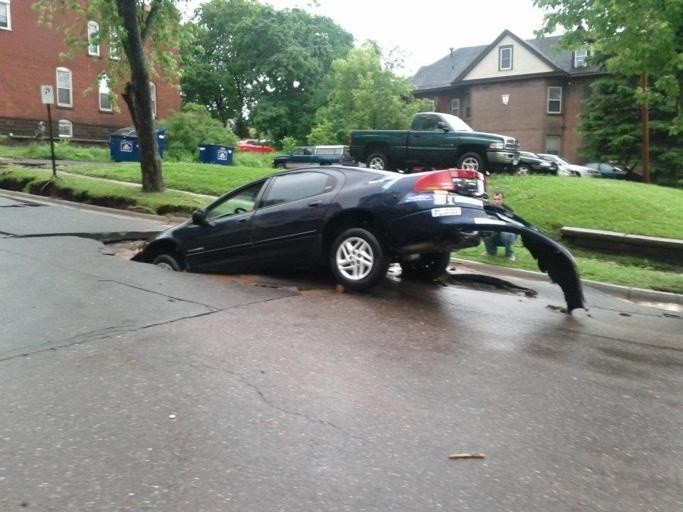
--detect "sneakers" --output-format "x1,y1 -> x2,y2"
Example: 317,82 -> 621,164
504,256 -> 516,261
481,251 -> 497,257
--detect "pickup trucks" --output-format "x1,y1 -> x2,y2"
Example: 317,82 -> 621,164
349,113 -> 519,172
274,146 -> 356,168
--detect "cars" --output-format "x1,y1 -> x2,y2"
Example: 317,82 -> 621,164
238,139 -> 276,152
519,151 -> 638,181
130,167 -> 585,313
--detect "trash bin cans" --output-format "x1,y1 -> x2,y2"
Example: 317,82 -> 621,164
197,143 -> 236,166
110,127 -> 167,163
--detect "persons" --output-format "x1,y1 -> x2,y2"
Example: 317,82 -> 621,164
481,191 -> 517,260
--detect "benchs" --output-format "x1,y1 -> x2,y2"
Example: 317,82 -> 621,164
560,226 -> 683,268
